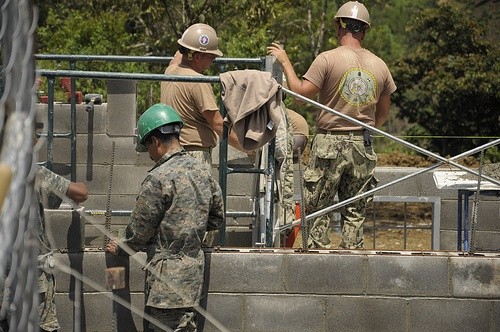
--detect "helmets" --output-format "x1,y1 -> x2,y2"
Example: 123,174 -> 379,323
333,0 -> 369,28
135,102 -> 184,152
176,22 -> 224,58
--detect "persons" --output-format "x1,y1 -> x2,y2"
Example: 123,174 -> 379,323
267,2 -> 399,248
0,119 -> 90,332
279,90 -> 310,249
103,104 -> 225,332
160,22 -> 256,249
